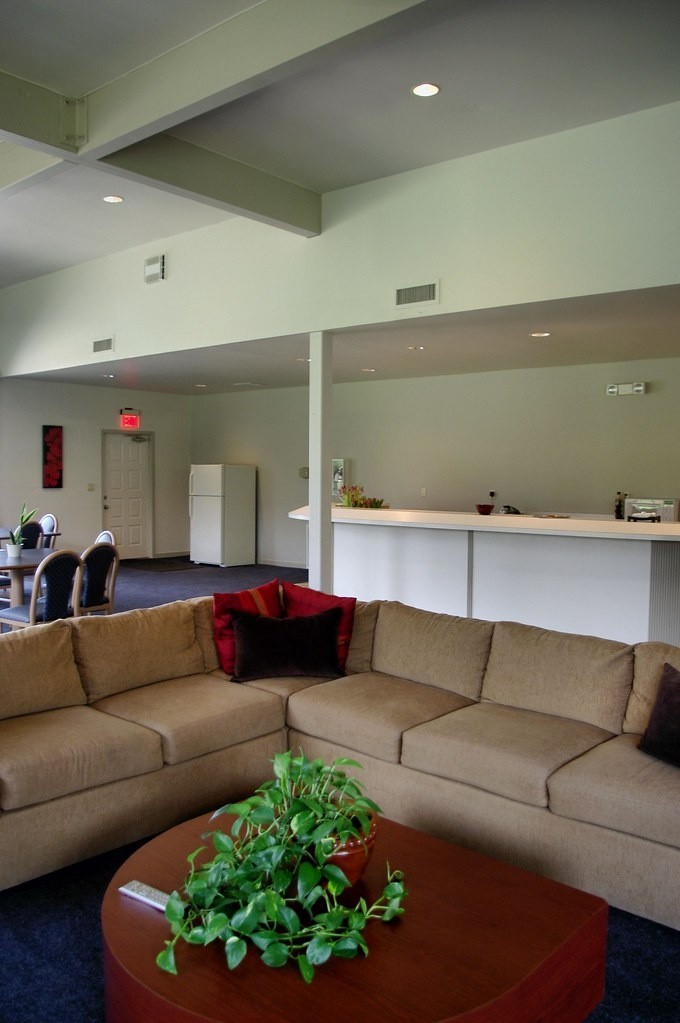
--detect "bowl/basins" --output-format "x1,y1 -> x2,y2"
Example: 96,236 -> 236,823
475,504 -> 494,515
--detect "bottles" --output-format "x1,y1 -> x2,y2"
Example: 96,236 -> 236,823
614,491 -> 624,519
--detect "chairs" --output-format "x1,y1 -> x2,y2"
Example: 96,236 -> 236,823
0,513 -> 120,633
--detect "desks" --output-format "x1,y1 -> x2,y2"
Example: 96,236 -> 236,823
101,775 -> 608,1023
0,549 -> 61,632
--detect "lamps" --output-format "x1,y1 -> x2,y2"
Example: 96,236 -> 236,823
605,381 -> 645,396
410,81 -> 441,97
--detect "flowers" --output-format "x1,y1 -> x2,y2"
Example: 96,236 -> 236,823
335,482 -> 384,509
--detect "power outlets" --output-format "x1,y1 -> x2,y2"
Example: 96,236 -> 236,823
489,490 -> 495,500
420,488 -> 426,497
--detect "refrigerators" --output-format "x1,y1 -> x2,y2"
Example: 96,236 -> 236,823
188,464 -> 256,568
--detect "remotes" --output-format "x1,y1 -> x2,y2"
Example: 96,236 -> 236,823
117,879 -> 170,912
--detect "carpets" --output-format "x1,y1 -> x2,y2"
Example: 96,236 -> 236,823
120,557 -> 202,573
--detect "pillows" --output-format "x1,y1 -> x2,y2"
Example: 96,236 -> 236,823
636,662 -> 680,770
213,577 -> 358,683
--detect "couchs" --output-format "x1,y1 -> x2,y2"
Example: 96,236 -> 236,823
0,584 -> 680,931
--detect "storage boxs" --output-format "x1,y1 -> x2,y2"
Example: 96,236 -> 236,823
625,498 -> 680,521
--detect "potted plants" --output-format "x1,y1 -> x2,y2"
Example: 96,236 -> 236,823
5,503 -> 37,558
154,743 -> 409,978
